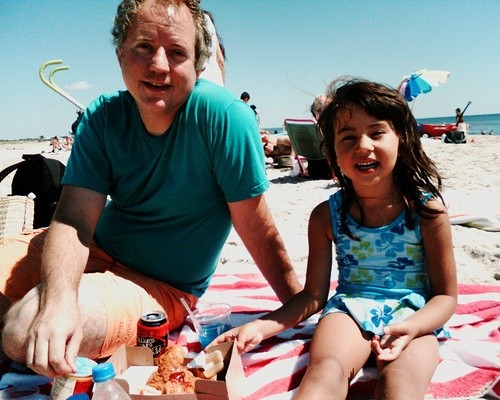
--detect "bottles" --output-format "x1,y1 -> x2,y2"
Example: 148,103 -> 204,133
91,362 -> 133,400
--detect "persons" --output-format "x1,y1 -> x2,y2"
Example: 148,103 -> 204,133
0,0 -> 303,379
442,107 -> 467,144
481,129 -> 494,135
47,135 -> 74,153
239,91 -> 297,172
204,76 -> 459,400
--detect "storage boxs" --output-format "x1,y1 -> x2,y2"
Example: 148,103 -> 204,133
106,338 -> 246,400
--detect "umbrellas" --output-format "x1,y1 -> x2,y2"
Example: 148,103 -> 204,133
397,70 -> 453,102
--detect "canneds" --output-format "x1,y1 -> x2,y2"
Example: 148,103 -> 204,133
50,356 -> 99,400
136,311 -> 168,359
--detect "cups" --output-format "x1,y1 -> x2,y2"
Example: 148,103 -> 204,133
186,303 -> 231,350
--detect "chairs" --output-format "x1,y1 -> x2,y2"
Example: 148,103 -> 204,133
284,119 -> 338,182
